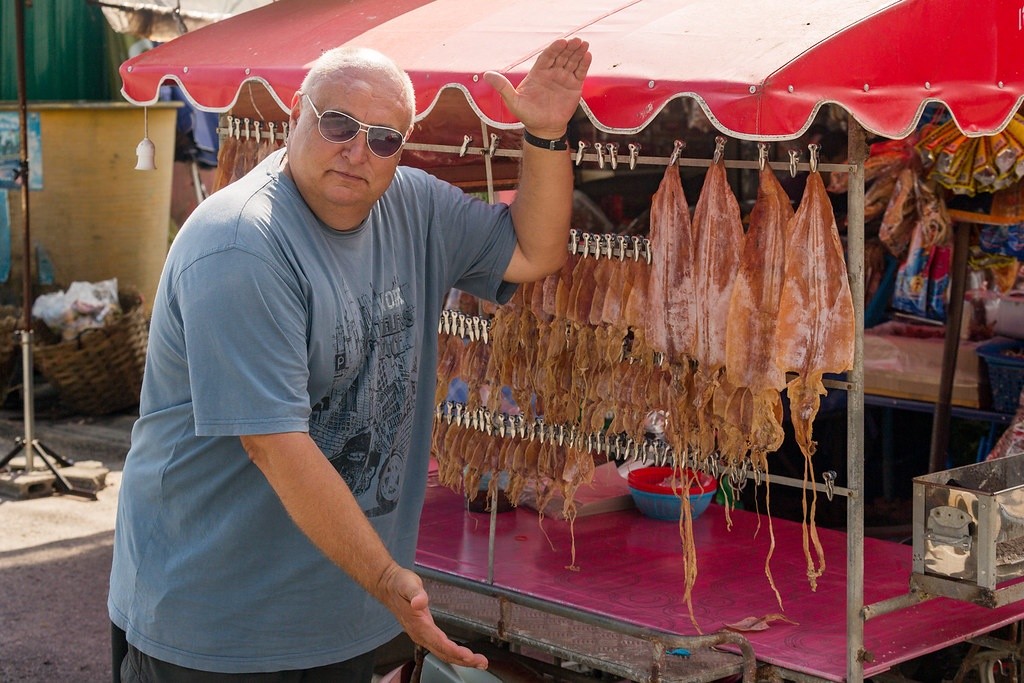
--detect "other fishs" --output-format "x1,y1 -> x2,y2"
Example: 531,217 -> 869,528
429,253 -> 786,573
210,135 -> 288,195
721,612 -> 800,632
689,157 -> 746,464
641,160 -> 705,636
723,161 -> 796,611
768,169 -> 856,593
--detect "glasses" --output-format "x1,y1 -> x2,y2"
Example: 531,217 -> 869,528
300,92 -> 408,158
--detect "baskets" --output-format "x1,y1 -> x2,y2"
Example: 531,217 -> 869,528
32,285 -> 149,411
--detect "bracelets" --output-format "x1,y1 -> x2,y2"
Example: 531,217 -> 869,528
523,125 -> 568,151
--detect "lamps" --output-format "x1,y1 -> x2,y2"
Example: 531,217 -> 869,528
132,105 -> 158,173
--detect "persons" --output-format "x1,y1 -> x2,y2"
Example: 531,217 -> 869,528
106,36 -> 593,682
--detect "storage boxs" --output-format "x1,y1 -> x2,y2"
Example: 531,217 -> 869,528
27,284 -> 148,415
846,318 -> 1000,410
973,340 -> 1024,415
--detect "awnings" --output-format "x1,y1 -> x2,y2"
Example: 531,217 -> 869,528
116,1 -> 1024,141
